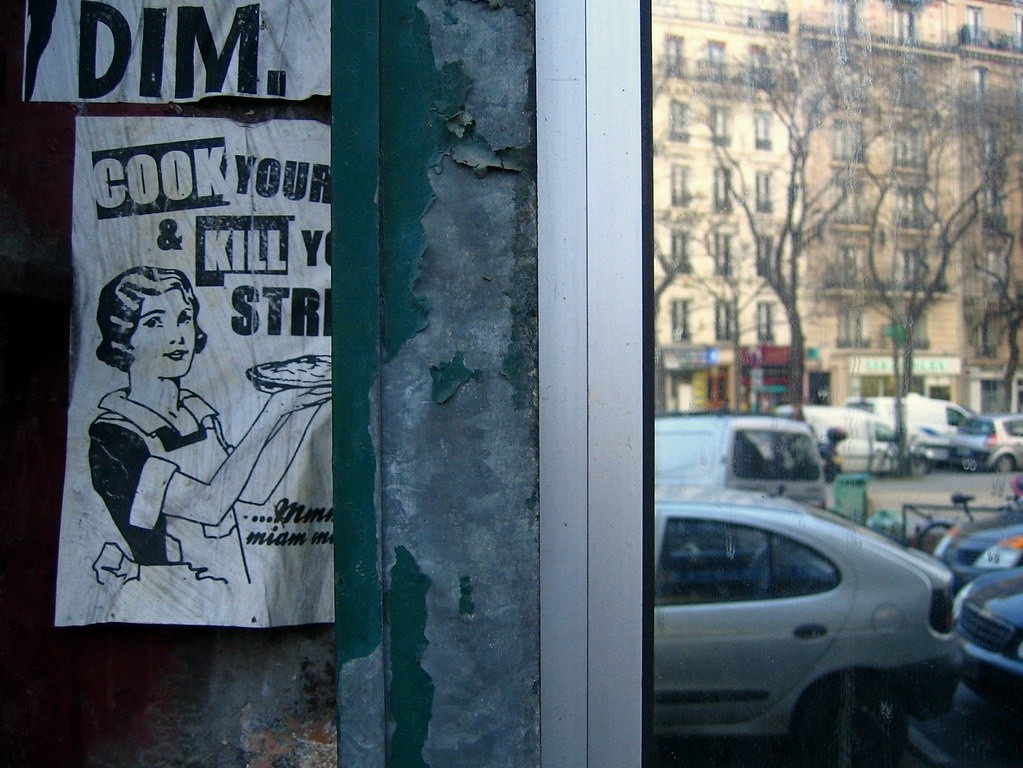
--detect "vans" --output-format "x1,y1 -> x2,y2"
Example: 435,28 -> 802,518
655,413 -> 827,510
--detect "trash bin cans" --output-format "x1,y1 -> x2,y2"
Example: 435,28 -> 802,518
865,508 -> 903,542
831,473 -> 870,525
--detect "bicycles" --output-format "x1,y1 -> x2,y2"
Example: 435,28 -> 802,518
917,489 -> 1023,553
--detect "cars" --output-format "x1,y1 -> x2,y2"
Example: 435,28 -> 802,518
932,506 -> 1023,716
655,481 -> 964,768
773,391 -> 1023,476
